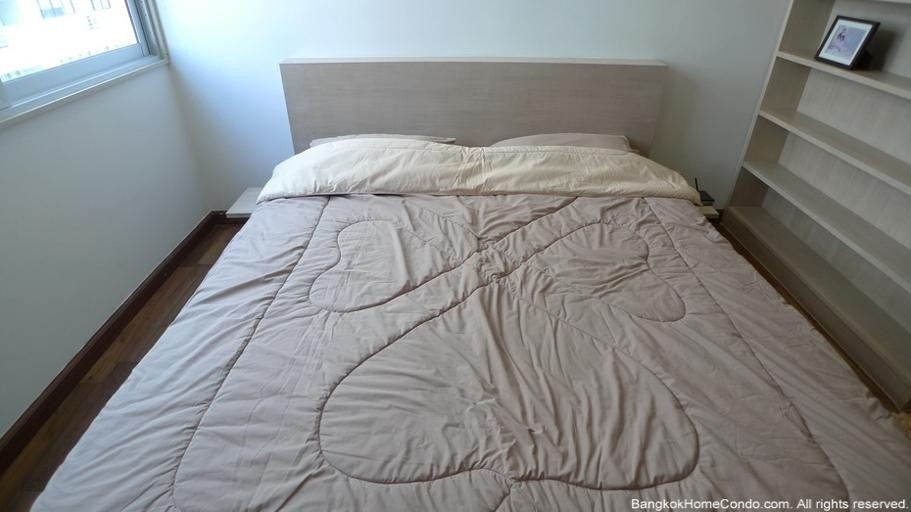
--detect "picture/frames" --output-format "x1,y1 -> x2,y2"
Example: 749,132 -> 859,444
815,13 -> 882,70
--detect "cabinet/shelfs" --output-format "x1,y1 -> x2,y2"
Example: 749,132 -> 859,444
720,0 -> 908,415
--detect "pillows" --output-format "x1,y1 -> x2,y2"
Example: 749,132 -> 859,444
308,132 -> 456,145
486,133 -> 643,155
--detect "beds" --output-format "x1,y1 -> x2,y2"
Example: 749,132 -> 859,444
22,58 -> 909,512
